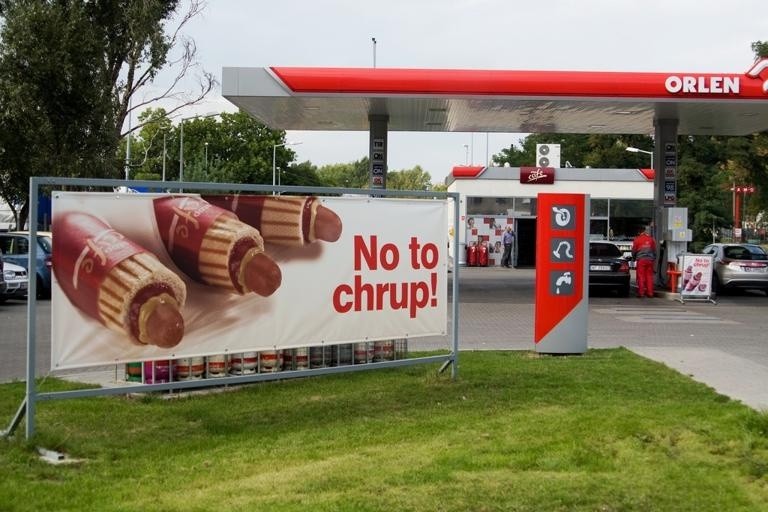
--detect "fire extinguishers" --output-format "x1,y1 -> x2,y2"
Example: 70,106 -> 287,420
479,242 -> 488,266
468,241 -> 477,267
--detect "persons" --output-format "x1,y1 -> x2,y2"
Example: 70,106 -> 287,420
494,241 -> 501,253
480,240 -> 488,247
467,218 -> 476,229
489,217 -> 501,230
500,224 -> 516,269
478,235 -> 482,244
632,225 -> 657,299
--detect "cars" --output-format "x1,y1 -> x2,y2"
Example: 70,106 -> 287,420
0,230 -> 52,300
588,243 -> 632,298
696,243 -> 767,296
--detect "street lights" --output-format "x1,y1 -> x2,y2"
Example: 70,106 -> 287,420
272,141 -> 303,195
625,147 -> 653,169
179,111 -> 220,194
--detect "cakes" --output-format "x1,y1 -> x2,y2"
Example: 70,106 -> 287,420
97,197 -> 343,349
